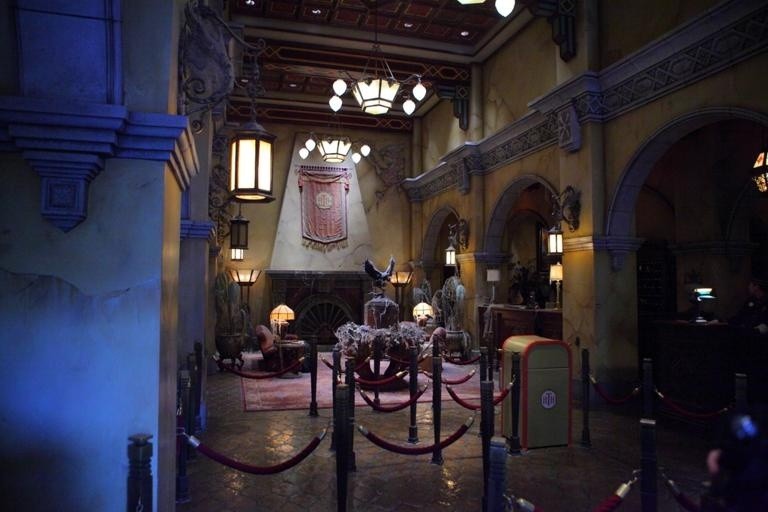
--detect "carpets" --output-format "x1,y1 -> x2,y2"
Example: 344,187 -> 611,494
238,350 -> 506,413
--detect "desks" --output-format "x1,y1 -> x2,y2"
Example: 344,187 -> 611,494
476,303 -> 564,374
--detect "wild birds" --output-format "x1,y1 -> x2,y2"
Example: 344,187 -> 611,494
364,255 -> 396,294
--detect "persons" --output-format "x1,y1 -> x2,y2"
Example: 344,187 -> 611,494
724,274 -> 767,331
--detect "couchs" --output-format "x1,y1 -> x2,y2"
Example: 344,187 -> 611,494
254,324 -> 314,374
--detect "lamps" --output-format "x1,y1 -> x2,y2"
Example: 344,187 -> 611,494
328,1 -> 427,116
389,270 -> 415,322
549,263 -> 563,310
298,68 -> 372,164
750,145 -> 768,197
444,218 -> 468,267
228,268 -> 262,310
546,185 -> 582,256
486,268 -> 502,304
177,0 -> 278,262
270,302 -> 295,338
412,299 -> 437,325
